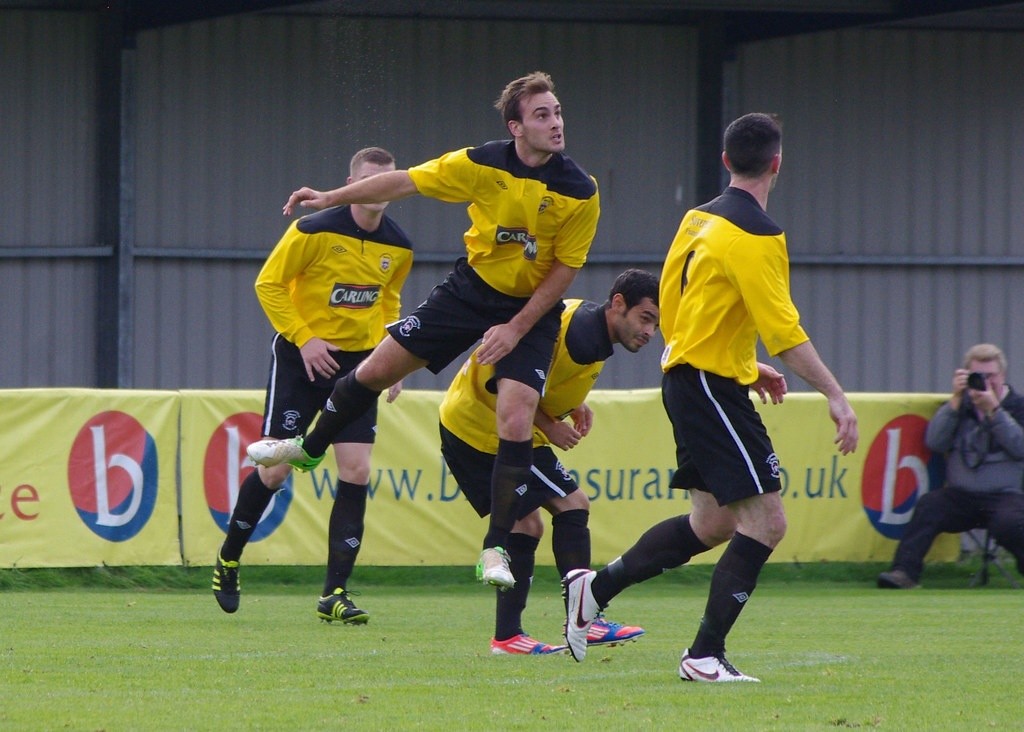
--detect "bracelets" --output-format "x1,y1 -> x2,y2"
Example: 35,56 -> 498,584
987,405 -> 1003,417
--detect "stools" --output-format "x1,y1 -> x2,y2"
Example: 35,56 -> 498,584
965,526 -> 1019,589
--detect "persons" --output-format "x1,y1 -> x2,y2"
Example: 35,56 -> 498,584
246,72 -> 600,589
212,147 -> 413,626
878,343 -> 1024,589
559,112 -> 859,683
439,268 -> 660,655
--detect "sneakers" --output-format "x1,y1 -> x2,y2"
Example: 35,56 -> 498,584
490,632 -> 569,656
678,647 -> 760,683
564,615 -> 646,647
212,546 -> 241,614
246,435 -> 327,473
315,586 -> 370,627
561,568 -> 609,662
475,546 -> 517,592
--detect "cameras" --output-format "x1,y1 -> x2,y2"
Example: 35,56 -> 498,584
967,370 -> 988,392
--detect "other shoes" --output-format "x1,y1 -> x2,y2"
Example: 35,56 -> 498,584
878,568 -> 916,589
